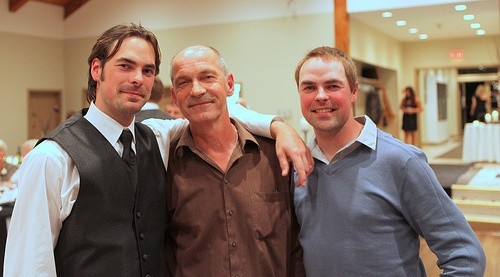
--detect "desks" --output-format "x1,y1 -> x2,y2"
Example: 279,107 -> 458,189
461,122 -> 500,166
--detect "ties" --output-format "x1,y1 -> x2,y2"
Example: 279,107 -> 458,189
119,129 -> 138,197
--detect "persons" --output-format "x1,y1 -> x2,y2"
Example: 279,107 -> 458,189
290,46 -> 486,277
401,87 -> 421,146
469,81 -> 500,123
0,21 -> 314,277
